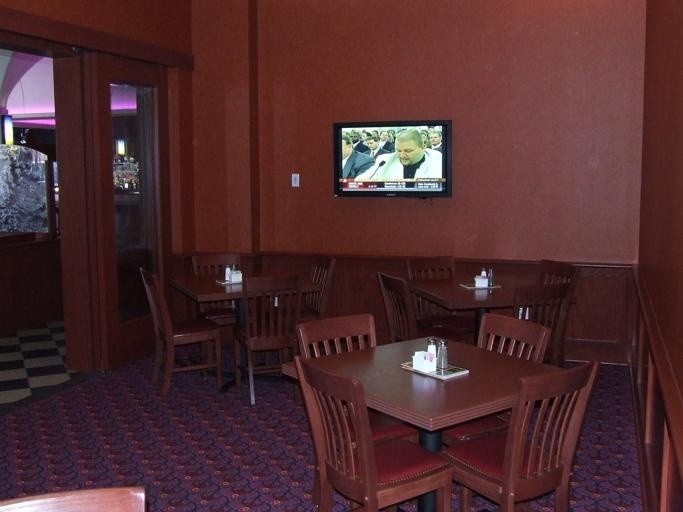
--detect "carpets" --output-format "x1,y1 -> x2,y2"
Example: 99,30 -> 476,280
0,342 -> 644,512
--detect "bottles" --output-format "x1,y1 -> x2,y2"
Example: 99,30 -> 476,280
488,267 -> 494,287
232,264 -> 237,275
437,340 -> 449,370
426,338 -> 437,359
224,264 -> 230,282
481,269 -> 486,276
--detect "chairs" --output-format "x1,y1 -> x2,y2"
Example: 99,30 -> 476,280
293,255 -> 334,361
139,266 -> 222,396
438,312 -> 550,446
377,273 -> 465,363
476,279 -> 576,357
232,271 -> 303,405
191,254 -> 243,325
438,357 -> 601,512
537,258 -> 582,336
407,257 -> 472,334
292,356 -> 453,512
293,313 -> 420,448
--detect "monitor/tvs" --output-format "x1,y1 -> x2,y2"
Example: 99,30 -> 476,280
332,120 -> 453,198
